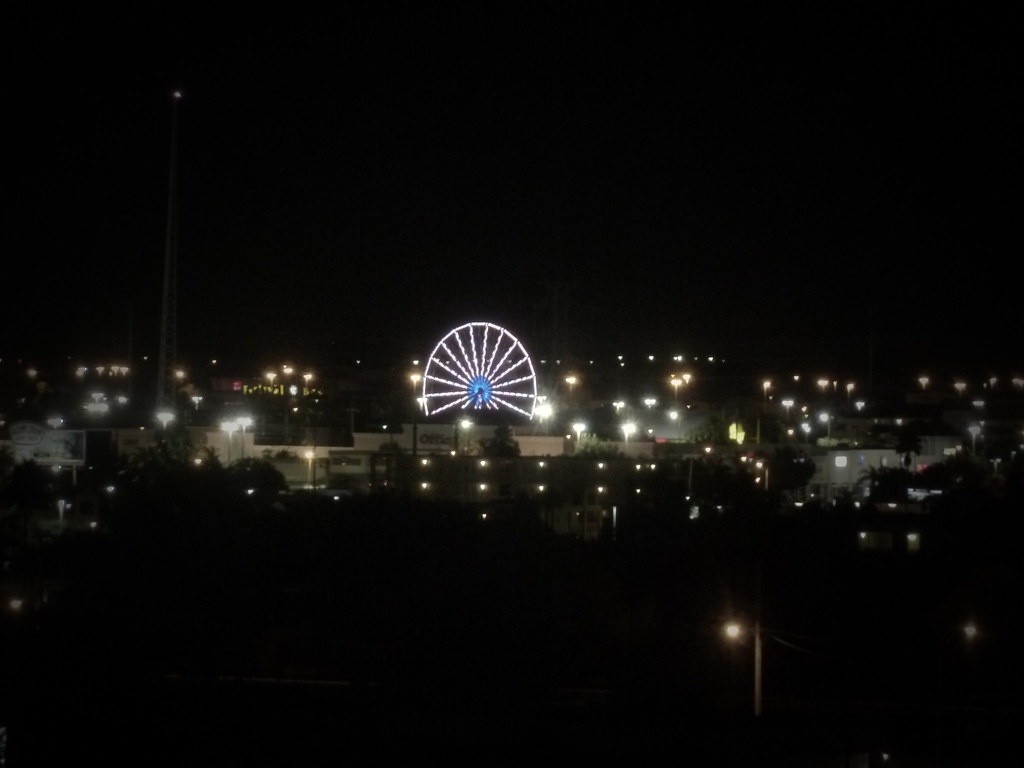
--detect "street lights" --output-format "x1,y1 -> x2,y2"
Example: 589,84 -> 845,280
623,422 -> 638,449
955,381 -> 966,416
222,421 -> 234,466
967,424 -> 979,454
818,412 -> 832,442
723,621 -> 762,720
238,418 -> 251,461
991,457 -> 1002,477
671,376 -> 683,411
568,374 -> 578,397
761,380 -> 773,412
408,372 -> 420,454
782,399 -> 794,421
461,419 -> 475,456
845,383 -> 855,409
754,458 -> 769,491
817,378 -> 830,397
304,449 -> 313,487
574,422 -> 587,450
920,378 -> 929,392
